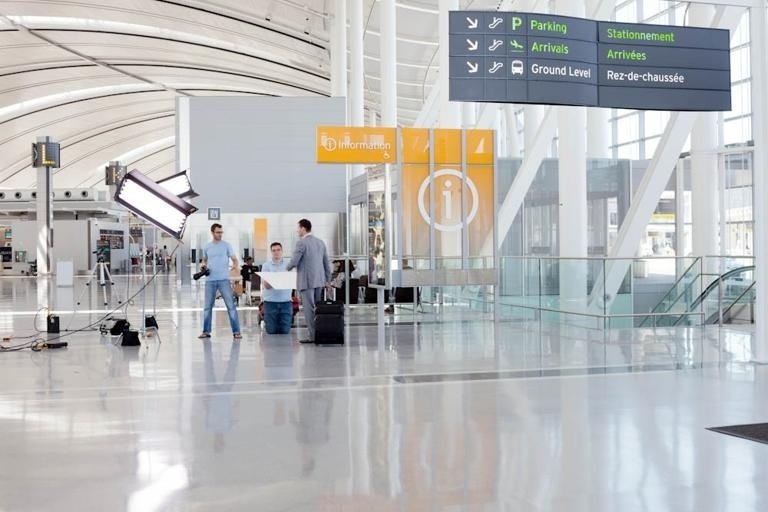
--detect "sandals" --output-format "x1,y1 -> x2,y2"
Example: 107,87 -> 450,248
234,334 -> 241,338
198,333 -> 210,338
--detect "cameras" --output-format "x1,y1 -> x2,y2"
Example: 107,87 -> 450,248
92,246 -> 109,262
194,266 -> 209,280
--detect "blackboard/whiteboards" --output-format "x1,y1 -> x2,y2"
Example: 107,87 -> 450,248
56,258 -> 73,287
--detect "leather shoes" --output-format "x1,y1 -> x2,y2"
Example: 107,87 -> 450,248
299,339 -> 314,343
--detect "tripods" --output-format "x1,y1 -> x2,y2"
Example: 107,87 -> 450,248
65,262 -> 127,331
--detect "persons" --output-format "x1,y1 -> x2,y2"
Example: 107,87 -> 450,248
332,251 -> 356,278
285,218 -> 330,344
255,240 -> 292,335
382,257 -> 413,314
161,245 -> 170,272
196,223 -> 243,339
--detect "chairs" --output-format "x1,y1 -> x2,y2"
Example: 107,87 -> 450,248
330,278 -> 421,305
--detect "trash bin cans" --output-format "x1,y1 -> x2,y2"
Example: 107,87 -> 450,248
162,256 -> 171,272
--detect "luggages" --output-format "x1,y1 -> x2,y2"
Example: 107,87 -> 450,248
314,286 -> 344,344
